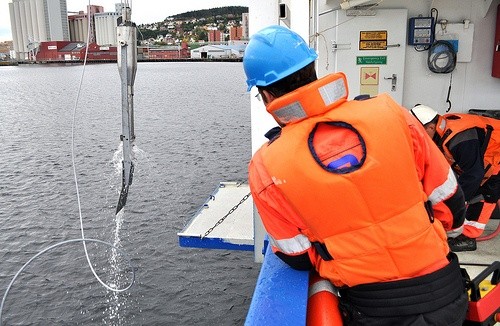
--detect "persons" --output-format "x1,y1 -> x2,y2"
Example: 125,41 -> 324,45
242,25 -> 469,326
409,104 -> 500,252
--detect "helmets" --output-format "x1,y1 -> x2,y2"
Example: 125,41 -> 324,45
243,25 -> 318,92
409,105 -> 437,126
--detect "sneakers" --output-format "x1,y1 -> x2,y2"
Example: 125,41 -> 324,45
447,233 -> 477,252
